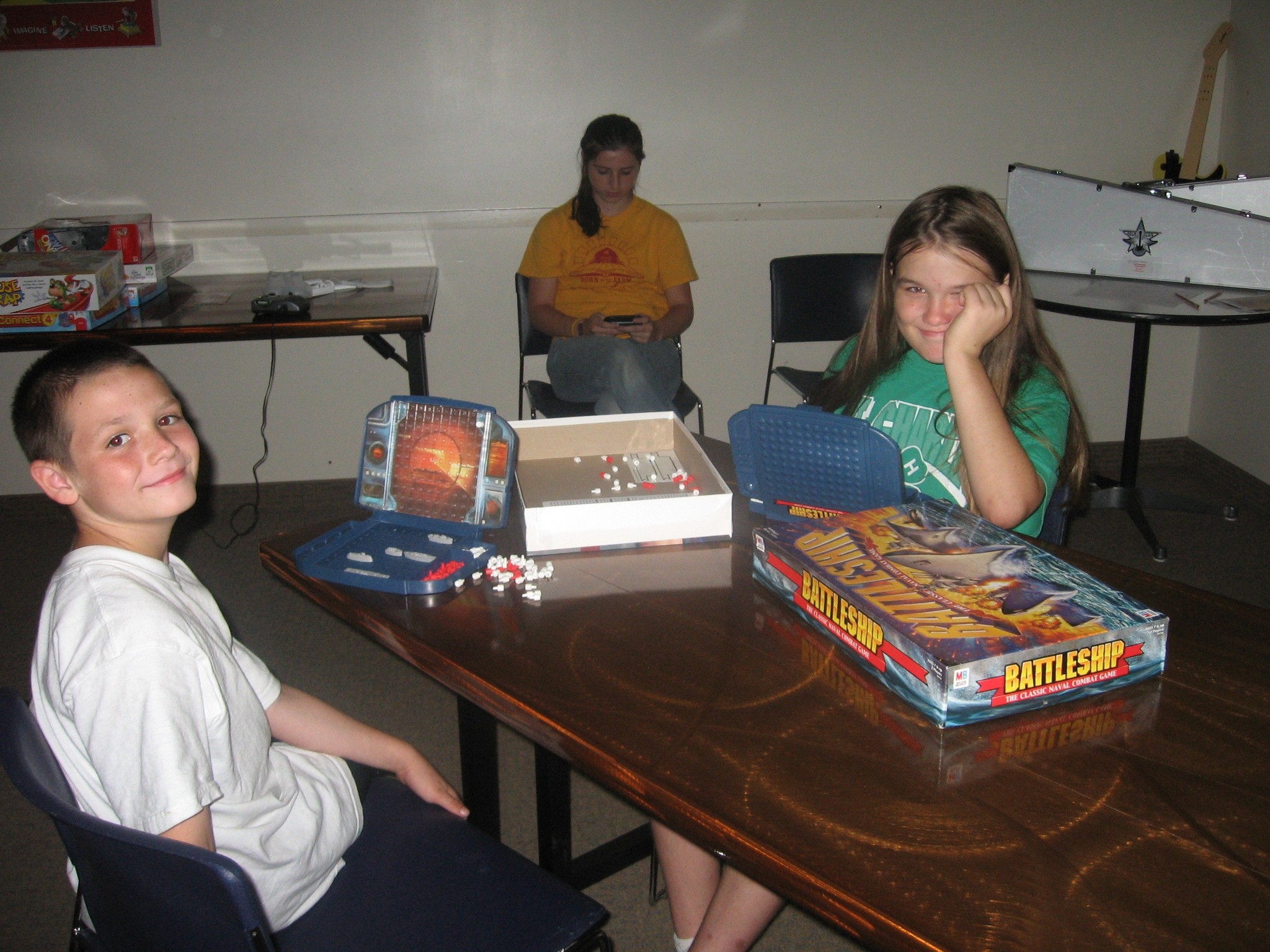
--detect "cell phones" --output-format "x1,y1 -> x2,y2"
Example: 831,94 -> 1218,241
604,315 -> 642,326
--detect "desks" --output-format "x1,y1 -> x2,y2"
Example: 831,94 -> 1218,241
0,266 -> 439,400
260,476 -> 1270,952
1013,272 -> 1270,563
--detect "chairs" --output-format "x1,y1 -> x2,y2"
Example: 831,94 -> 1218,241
516,273 -> 704,442
0,688 -> 610,952
647,410 -> 1073,910
763,253 -> 884,412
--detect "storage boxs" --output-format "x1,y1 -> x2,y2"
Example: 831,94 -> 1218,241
0,196 -> 195,331
499,411 -> 733,557
752,501 -> 1169,728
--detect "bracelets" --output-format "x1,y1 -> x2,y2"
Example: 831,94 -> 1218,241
571,317 -> 585,336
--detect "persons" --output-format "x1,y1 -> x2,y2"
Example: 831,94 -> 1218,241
650,186 -> 1096,952
513,114 -> 699,419
0,340 -> 468,952
